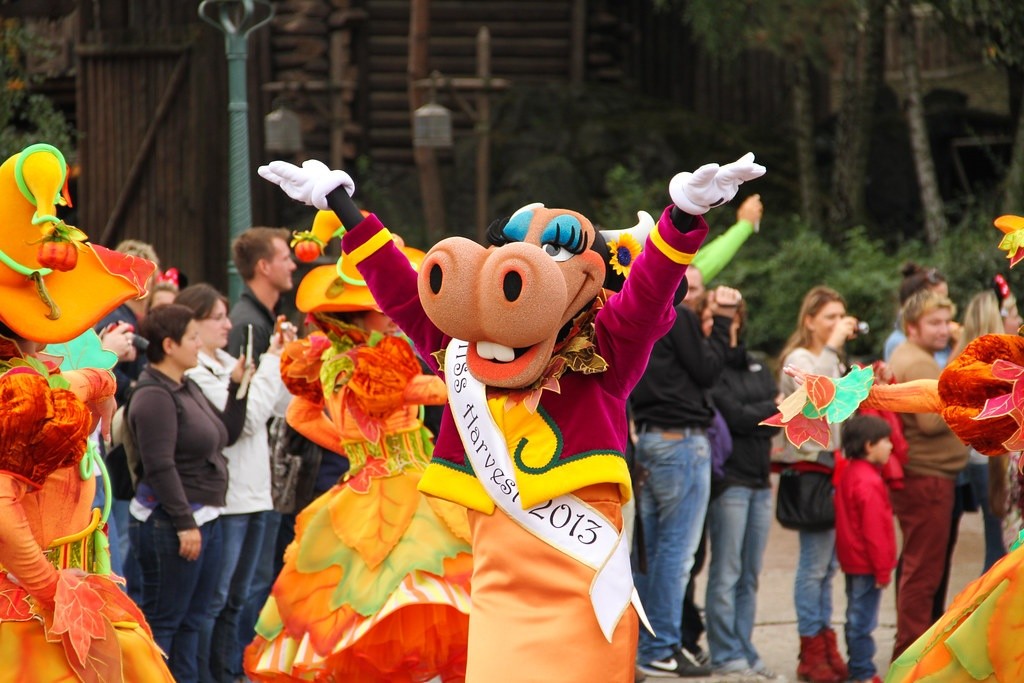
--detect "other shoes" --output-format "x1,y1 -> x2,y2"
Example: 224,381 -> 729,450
755,668 -> 789,682
711,665 -> 768,682
861,675 -> 883,683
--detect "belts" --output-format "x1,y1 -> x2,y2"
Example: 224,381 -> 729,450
635,422 -> 704,433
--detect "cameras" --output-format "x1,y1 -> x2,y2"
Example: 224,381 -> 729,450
108,323 -> 151,354
852,322 -> 869,336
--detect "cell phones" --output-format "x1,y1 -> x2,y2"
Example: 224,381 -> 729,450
243,323 -> 253,367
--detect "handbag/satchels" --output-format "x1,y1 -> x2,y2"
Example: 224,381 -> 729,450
268,416 -> 323,515
774,469 -> 837,529
710,413 -> 735,476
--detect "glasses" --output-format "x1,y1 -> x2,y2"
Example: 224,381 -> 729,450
913,268 -> 944,295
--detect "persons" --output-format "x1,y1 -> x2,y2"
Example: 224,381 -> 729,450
0,143 -> 176,683
273,209 -> 426,572
95,227 -> 351,683
627,194 -> 1024,683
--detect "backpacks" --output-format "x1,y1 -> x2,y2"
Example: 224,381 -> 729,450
105,380 -> 182,501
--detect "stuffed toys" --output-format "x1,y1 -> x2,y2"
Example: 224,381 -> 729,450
257,151 -> 766,683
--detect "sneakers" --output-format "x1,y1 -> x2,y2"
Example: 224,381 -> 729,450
682,629 -> 712,669
635,645 -> 714,678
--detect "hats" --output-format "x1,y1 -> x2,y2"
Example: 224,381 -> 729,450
0,144 -> 156,343
290,208 -> 426,313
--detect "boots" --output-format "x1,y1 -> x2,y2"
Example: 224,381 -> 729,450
820,627 -> 851,679
797,634 -> 840,682
